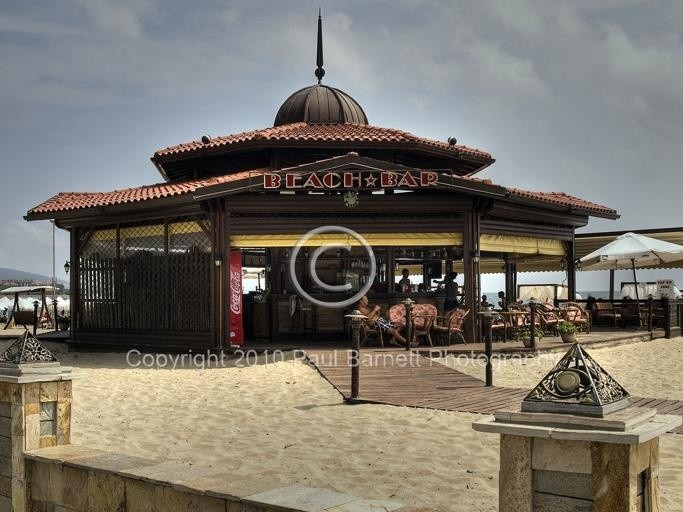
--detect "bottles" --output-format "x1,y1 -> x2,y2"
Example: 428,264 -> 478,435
350,256 -> 382,283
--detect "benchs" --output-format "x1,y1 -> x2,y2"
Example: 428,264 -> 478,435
11,311 -> 34,331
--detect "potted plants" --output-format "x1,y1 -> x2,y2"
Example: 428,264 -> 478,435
513,324 -> 545,348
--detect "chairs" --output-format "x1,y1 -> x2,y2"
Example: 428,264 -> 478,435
434,305 -> 471,346
489,302 -> 592,343
360,317 -> 387,348
287,295 -> 317,340
591,299 -> 664,328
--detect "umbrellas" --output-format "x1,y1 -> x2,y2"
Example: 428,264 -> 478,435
575,232 -> 683,328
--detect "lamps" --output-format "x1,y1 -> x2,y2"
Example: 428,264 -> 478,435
63,260 -> 72,273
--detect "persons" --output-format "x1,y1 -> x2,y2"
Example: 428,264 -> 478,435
481,295 -> 489,307
498,291 -> 507,310
399,269 -> 411,293
438,272 -> 460,312
355,293 -> 419,348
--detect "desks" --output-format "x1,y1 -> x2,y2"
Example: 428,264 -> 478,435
403,314 -> 436,347
